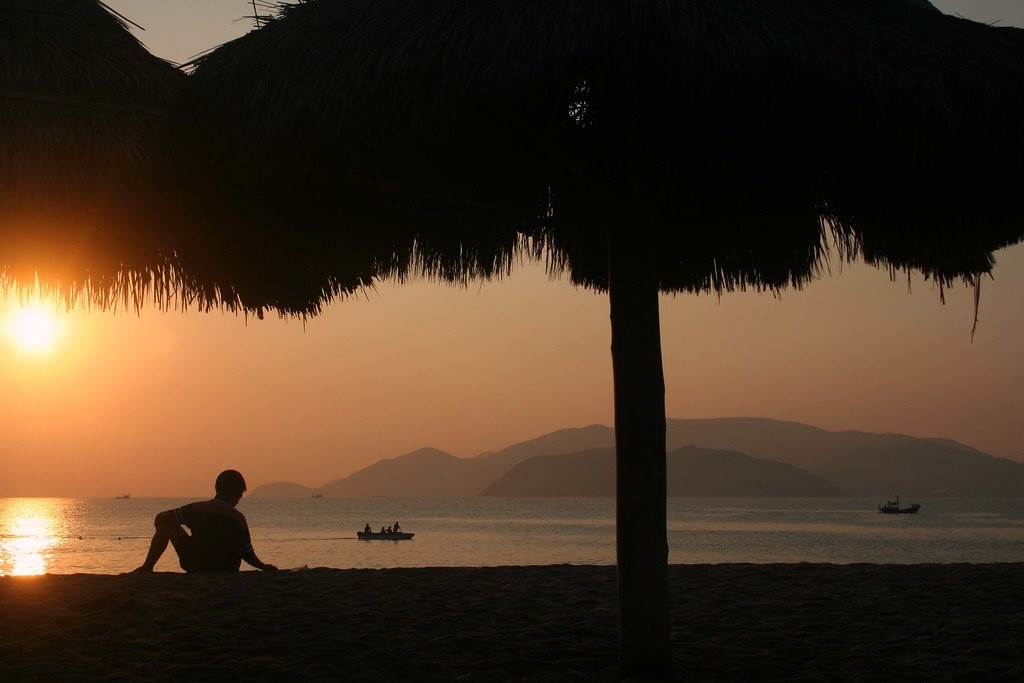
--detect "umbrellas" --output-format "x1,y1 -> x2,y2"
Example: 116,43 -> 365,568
172,0 -> 1024,683
0,0 -> 189,316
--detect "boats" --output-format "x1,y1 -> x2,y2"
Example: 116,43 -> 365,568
357,531 -> 415,541
877,493 -> 922,514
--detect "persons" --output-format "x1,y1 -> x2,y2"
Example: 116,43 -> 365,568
131,469 -> 279,575
365,524 -> 372,533
381,522 -> 400,533
